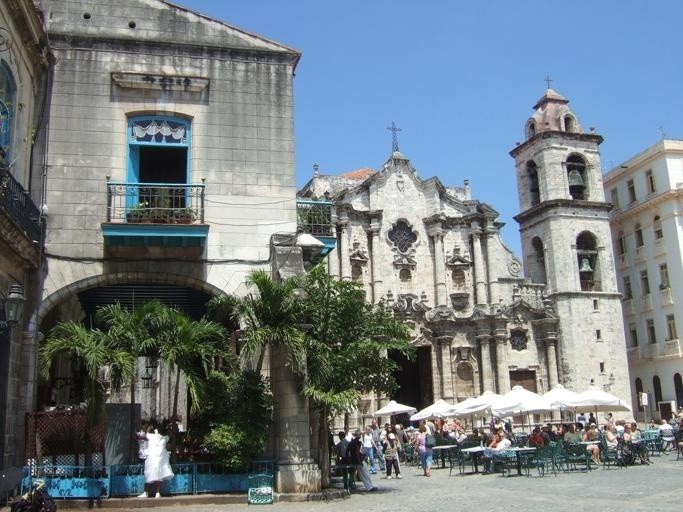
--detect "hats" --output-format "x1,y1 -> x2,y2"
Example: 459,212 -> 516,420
352,429 -> 364,438
388,433 -> 396,440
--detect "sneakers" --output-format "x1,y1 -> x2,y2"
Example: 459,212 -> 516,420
367,467 -> 432,491
137,491 -> 162,499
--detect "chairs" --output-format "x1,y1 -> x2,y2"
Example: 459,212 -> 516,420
246,457 -> 274,506
401,422 -> 664,480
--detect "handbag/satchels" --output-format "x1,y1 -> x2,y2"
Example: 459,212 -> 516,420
426,434 -> 436,447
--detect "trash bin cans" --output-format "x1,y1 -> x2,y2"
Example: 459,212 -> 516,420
248,473 -> 274,505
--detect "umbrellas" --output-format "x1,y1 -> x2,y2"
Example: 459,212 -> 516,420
369,383 -> 630,433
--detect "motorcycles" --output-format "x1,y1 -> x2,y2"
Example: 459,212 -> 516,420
7,478 -> 56,512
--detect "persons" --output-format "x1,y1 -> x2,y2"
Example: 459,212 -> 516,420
529,414 -> 641,465
336,417 -> 467,491
657,414 -> 682,451
137,419 -> 175,498
479,415 -> 513,459
